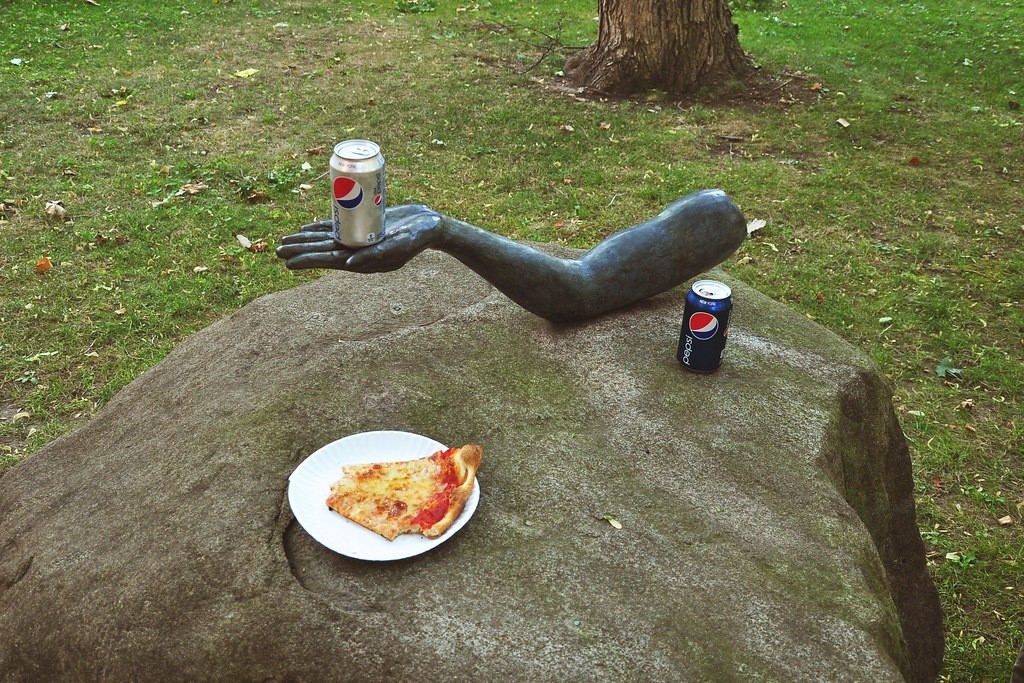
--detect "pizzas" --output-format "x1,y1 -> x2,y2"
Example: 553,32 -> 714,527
326,441 -> 481,540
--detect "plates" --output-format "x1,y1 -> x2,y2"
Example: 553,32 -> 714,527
288,430 -> 480,561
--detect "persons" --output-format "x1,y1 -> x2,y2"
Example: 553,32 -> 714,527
278,189 -> 749,320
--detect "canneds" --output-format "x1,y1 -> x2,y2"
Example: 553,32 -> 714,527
329,139 -> 386,249
677,280 -> 735,373
